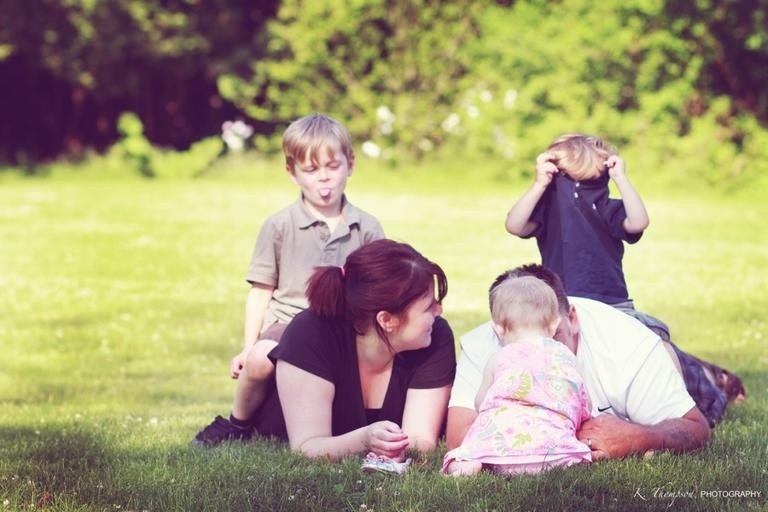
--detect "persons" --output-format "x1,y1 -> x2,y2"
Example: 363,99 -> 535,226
190,113 -> 746,477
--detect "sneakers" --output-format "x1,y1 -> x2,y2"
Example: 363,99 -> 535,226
192,415 -> 253,447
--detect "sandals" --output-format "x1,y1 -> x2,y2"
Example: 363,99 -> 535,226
691,357 -> 748,405
